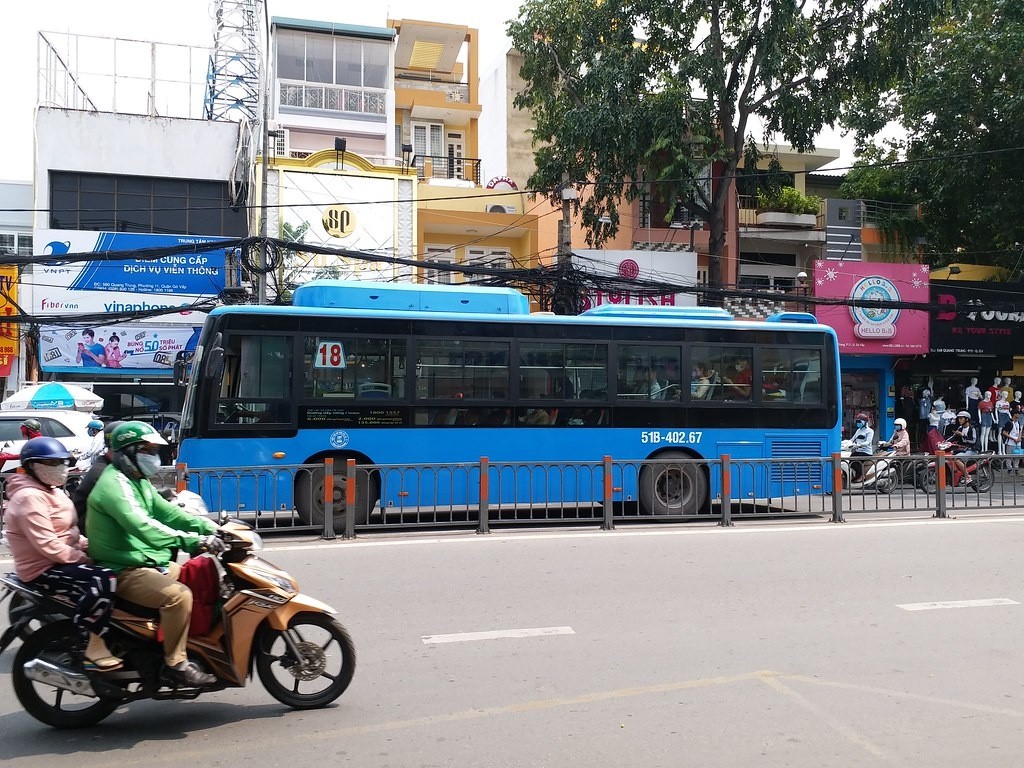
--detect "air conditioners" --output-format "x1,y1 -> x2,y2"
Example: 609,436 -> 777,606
486,203 -> 517,214
273,128 -> 292,157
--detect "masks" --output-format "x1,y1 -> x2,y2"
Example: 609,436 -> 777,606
857,423 -> 866,429
87,428 -> 94,436
894,425 -> 901,431
131,451 -> 162,476
31,462 -> 70,487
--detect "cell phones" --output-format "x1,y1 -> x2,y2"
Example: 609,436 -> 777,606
78,343 -> 83,346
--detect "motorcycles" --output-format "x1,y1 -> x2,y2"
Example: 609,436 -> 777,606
0,510 -> 357,731
873,435 -> 930,489
0,440 -> 81,493
826,435 -> 899,496
920,441 -> 1000,494
8,488 -> 209,642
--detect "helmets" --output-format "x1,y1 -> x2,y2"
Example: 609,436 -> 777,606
109,421 -> 169,451
20,436 -> 73,467
956,411 -> 971,419
104,420 -> 127,445
85,419 -> 104,431
856,413 -> 869,422
893,418 -> 907,429
20,419 -> 42,433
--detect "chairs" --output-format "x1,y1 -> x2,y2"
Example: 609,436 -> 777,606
445,406 -> 606,426
657,378 -> 669,399
703,371 -> 719,401
791,358 -> 812,403
357,383 -> 392,396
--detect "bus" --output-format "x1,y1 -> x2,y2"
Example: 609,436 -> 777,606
172,279 -> 843,532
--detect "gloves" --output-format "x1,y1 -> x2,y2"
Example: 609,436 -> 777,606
196,532 -> 226,554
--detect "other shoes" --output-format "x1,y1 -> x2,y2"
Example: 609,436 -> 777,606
852,475 -> 867,483
1006,469 -> 1023,477
958,475 -> 973,486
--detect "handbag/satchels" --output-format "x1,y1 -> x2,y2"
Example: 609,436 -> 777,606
178,552 -> 220,638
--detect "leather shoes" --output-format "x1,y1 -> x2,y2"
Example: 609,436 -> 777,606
159,661 -> 217,686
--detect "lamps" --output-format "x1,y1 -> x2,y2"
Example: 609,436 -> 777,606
947,266 -> 962,282
840,233 -> 859,259
401,142 -> 412,175
903,236 -> 929,264
335,136 -> 346,170
590,216 -> 612,249
659,221 -> 684,253
797,271 -> 807,287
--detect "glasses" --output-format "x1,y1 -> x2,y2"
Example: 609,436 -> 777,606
28,457 -> 70,467
133,447 -> 161,456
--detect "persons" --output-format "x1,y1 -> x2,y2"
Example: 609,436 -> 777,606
76,329 -> 105,367
73,420 -> 127,538
4,437 -> 125,671
85,420 -> 226,686
73,420 -> 106,465
724,356 -> 753,401
965,377 -> 1024,455
691,362 -> 711,399
630,364 -> 661,400
0,419 -> 42,460
773,352 -> 800,391
1002,409 -> 1022,474
888,417 -> 910,455
518,394 -> 550,424
850,413 -> 874,482
900,384 -> 957,434
104,332 -> 127,367
946,411 -> 978,485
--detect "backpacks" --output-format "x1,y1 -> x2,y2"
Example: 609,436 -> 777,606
1001,421 -> 1022,443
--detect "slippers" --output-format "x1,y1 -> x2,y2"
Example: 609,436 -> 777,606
82,654 -> 125,671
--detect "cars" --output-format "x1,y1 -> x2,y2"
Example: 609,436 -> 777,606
0,410 -> 99,485
